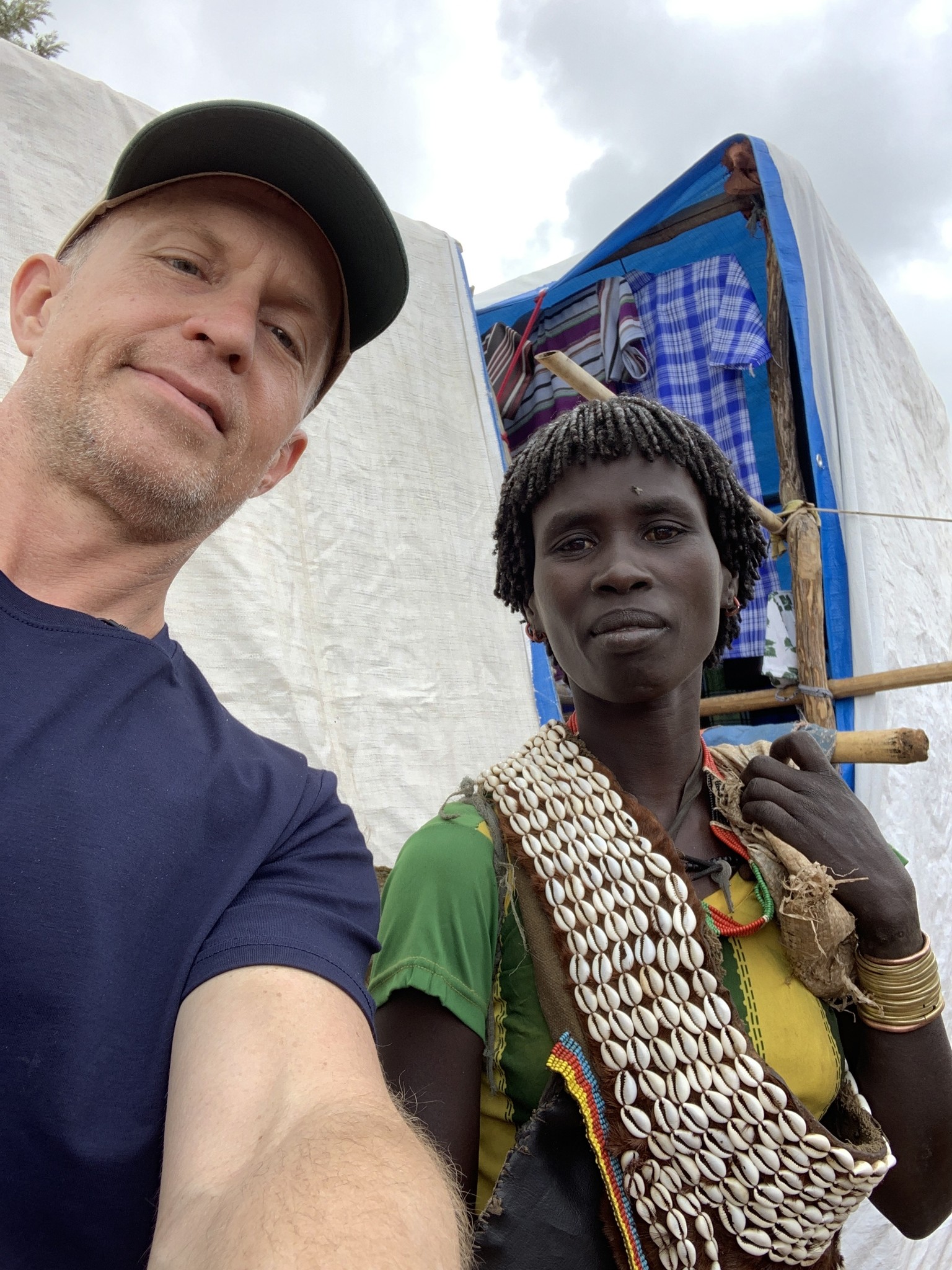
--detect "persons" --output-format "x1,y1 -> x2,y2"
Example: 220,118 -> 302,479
1,97 -> 474,1270
359,394 -> 952,1270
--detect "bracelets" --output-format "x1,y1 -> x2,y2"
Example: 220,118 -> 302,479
852,929 -> 947,1033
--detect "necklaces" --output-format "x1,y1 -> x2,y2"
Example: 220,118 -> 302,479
561,706 -> 776,936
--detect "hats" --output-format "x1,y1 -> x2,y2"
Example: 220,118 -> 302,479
58,97 -> 412,409
